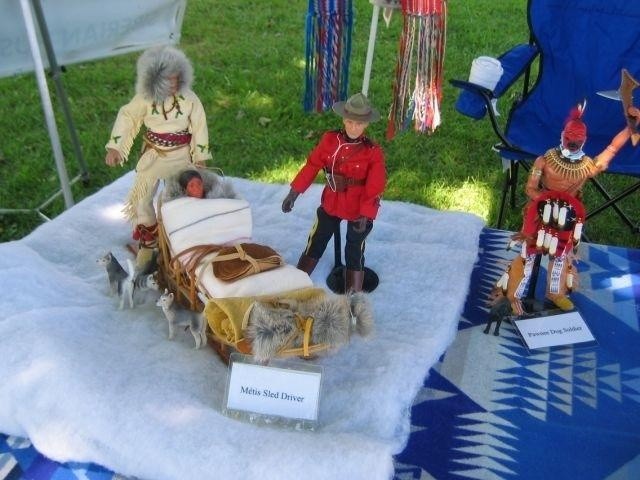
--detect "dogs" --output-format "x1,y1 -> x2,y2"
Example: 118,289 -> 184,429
155,287 -> 208,351
483,294 -> 513,336
95,250 -> 136,311
117,270 -> 161,302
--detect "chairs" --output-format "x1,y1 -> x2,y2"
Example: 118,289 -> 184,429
449,0 -> 640,241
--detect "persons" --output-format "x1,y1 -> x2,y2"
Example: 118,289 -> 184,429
101,44 -> 214,273
280,91 -> 386,299
505,97 -> 640,317
179,170 -> 203,199
